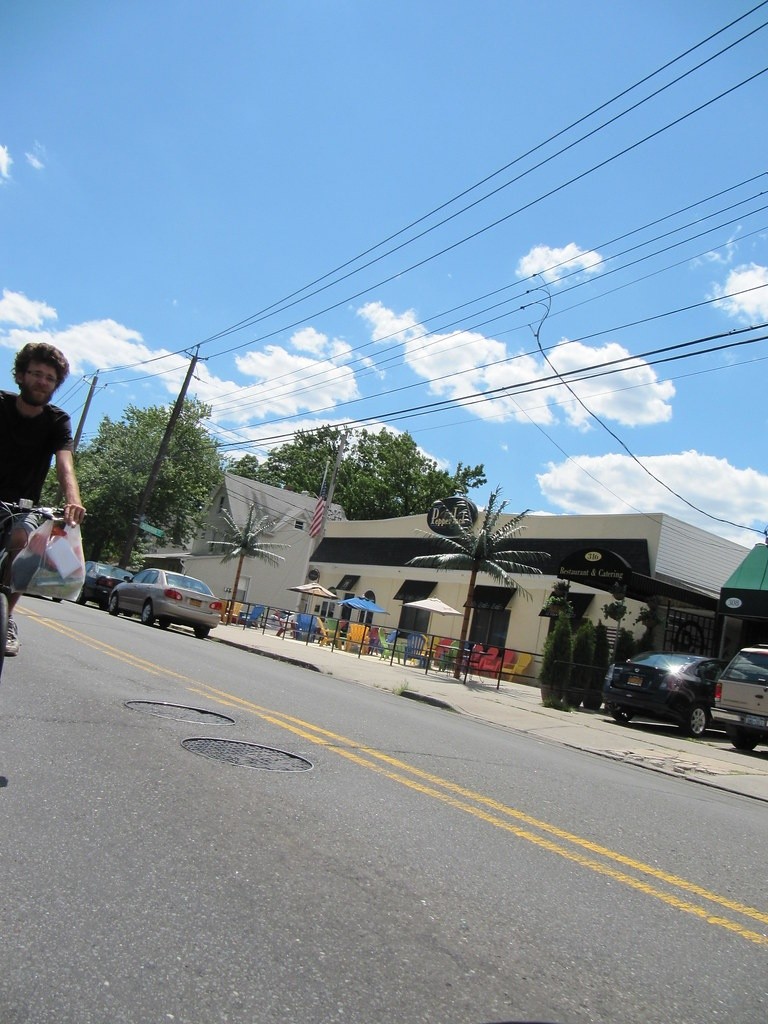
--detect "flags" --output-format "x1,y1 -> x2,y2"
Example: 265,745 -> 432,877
308,480 -> 326,538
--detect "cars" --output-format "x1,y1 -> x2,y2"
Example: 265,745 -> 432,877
601,651 -> 730,738
108,568 -> 222,639
77,561 -> 134,616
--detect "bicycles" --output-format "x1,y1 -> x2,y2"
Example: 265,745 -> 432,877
1,499 -> 92,676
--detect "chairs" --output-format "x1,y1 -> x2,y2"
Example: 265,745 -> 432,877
224,599 -> 533,681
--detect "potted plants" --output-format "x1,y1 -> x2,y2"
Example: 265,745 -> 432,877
538,581 -> 661,710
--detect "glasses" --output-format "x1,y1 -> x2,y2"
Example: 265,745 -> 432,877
24,370 -> 58,384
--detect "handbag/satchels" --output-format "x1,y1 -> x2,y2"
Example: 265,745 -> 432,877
11,518 -> 90,604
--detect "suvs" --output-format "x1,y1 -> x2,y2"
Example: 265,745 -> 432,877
711,644 -> 768,750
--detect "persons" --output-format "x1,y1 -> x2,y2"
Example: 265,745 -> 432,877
0,343 -> 85,657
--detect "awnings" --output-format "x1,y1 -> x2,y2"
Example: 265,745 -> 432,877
717,543 -> 768,618
538,591 -> 595,619
463,585 -> 517,612
393,580 -> 438,602
336,575 -> 360,591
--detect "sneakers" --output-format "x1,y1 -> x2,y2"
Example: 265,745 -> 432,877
5,619 -> 20,656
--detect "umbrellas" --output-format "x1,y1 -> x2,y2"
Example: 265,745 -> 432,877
399,595 -> 464,634
337,593 -> 391,623
286,581 -> 338,615
